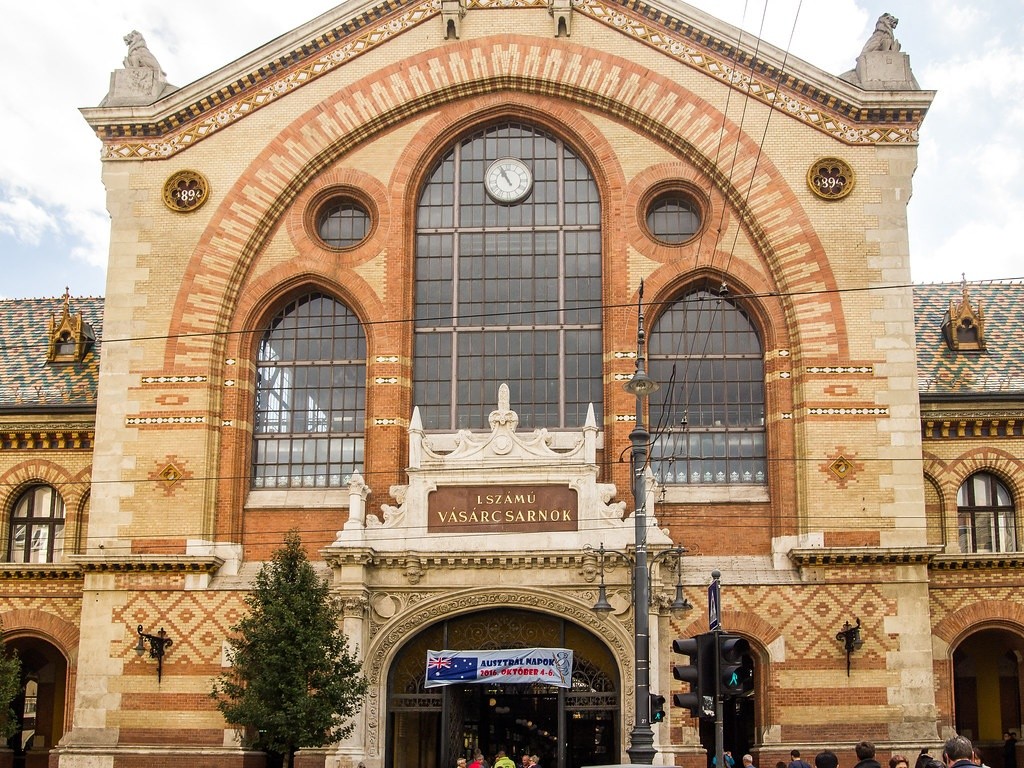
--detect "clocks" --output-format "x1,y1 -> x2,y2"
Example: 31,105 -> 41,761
483,156 -> 535,207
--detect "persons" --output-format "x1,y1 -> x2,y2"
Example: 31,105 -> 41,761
468,754 -> 484,768
942,736 -> 982,768
889,755 -> 909,768
466,749 -> 489,768
457,758 -> 467,768
915,748 -> 947,768
972,747 -> 991,768
788,750 -> 812,768
520,755 -> 530,768
528,755 -> 542,768
776,762 -> 787,768
491,755 -> 500,768
853,741 -> 881,768
1003,732 -> 1017,768
814,751 -> 839,768
743,754 -> 756,768
713,751 -> 734,768
494,751 -> 516,768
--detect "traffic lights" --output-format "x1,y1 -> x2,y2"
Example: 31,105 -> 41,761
650,694 -> 666,722
718,634 -> 750,696
673,632 -> 714,719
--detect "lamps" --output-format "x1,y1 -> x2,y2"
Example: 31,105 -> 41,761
133,625 -> 173,684
835,618 -> 865,677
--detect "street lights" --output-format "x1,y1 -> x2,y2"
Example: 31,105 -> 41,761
589,277 -> 692,765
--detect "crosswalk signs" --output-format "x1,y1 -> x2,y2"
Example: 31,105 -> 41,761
707,579 -> 719,632
